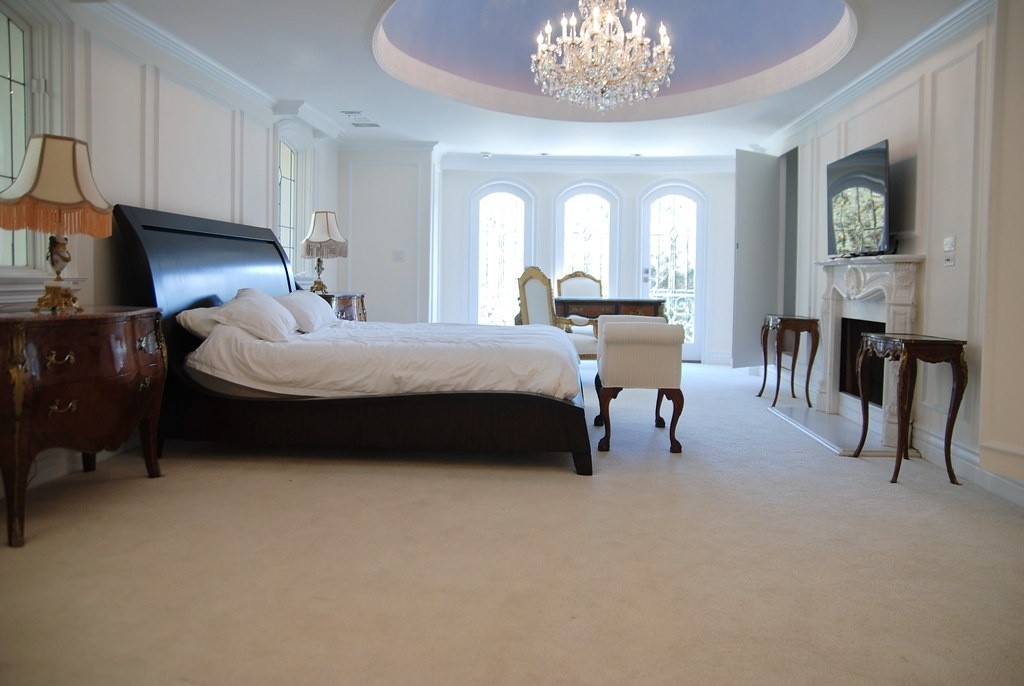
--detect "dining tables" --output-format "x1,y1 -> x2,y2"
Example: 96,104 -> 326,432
554,296 -> 667,319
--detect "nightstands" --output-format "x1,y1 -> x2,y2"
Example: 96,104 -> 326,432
0,306 -> 171,549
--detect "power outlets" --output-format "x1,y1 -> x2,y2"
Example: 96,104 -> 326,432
943,252 -> 955,267
943,236 -> 956,251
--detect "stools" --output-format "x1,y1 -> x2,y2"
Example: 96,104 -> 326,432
852,331 -> 968,486
755,314 -> 820,409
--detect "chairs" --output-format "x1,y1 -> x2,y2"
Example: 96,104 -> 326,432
517,265 -> 598,359
557,271 -> 603,338
592,314 -> 685,454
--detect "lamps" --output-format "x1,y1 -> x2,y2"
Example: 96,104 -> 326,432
0,132 -> 115,315
299,210 -> 349,295
531,0 -> 676,113
480,152 -> 492,158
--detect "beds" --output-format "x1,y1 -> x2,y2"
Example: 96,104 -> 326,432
112,203 -> 593,476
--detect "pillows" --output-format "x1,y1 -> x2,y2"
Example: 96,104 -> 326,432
176,305 -> 222,340
275,289 -> 338,332
210,287 -> 301,343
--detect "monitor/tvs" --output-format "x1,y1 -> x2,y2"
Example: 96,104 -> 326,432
826,138 -> 890,256
